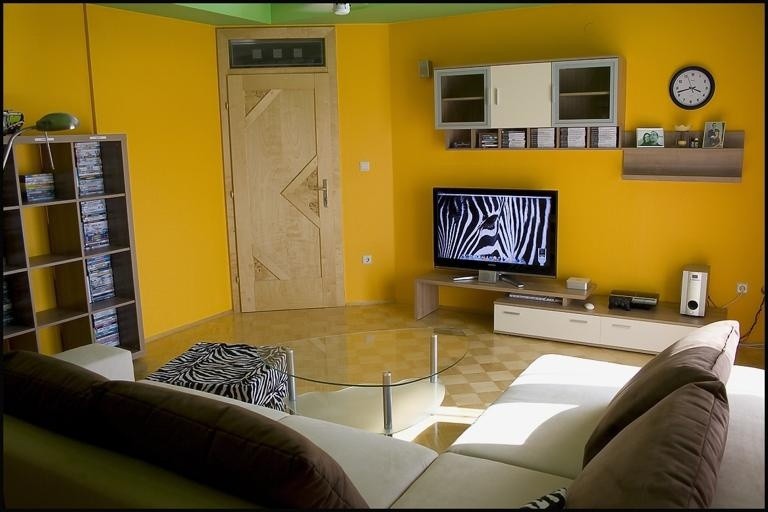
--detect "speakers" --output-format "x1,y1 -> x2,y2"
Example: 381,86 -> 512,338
680,265 -> 710,316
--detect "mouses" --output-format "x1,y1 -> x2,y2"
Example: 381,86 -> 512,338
584,302 -> 595,310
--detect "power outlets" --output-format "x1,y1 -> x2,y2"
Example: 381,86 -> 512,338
736,281 -> 748,293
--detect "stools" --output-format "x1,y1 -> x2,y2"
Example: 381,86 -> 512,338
144,340 -> 288,412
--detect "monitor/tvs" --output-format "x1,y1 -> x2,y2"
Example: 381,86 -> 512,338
433,187 -> 558,287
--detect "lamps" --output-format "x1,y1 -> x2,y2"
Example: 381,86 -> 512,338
3,112 -> 79,170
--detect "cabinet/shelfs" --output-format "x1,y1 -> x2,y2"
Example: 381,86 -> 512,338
414,269 -> 729,356
622,130 -> 745,183
432,54 -> 625,150
2,133 -> 146,362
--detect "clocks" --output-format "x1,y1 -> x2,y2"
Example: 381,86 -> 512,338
669,66 -> 715,111
